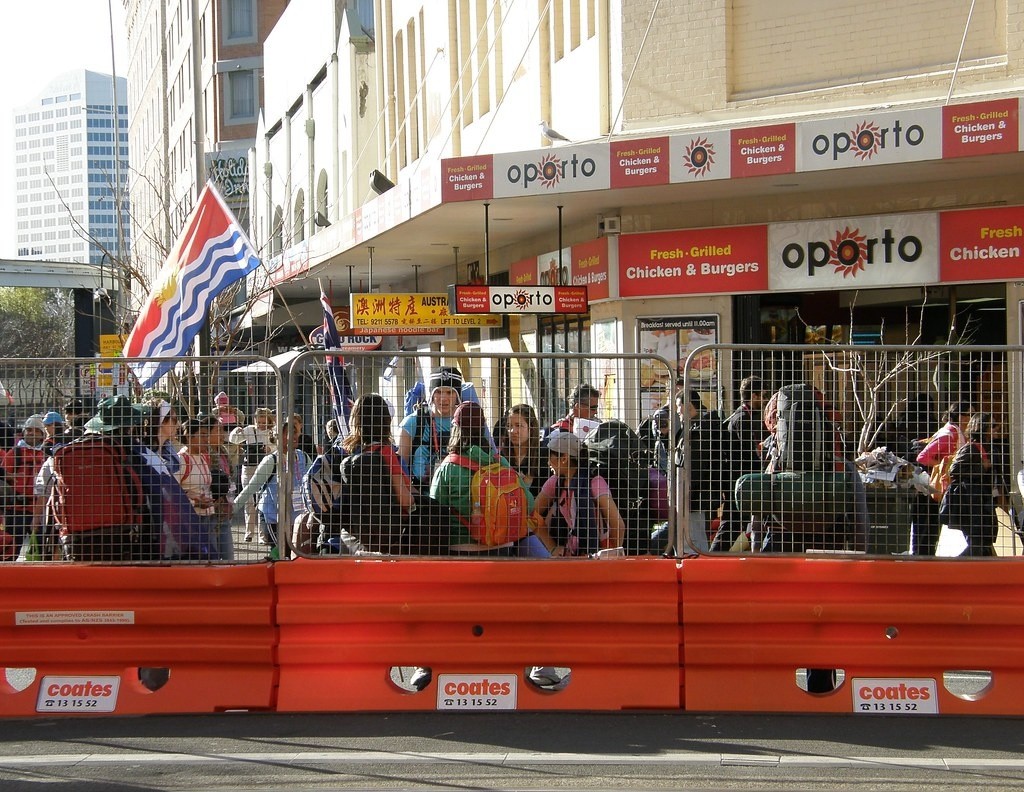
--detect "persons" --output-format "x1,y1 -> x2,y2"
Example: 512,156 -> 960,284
1,368 -> 1024,561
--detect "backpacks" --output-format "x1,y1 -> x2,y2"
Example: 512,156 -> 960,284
302,440 -> 347,522
53,433 -> 144,533
770,385 -> 844,473
446,452 -> 529,547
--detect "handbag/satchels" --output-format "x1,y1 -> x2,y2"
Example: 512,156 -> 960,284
939,444 -> 982,515
26,531 -> 41,561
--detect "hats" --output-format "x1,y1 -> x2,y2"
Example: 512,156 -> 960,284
547,432 -> 581,456
429,366 -> 462,407
42,411 -> 62,424
214,392 -> 229,404
22,414 -> 45,435
84,394 -> 150,432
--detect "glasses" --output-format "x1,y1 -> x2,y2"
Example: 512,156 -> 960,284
169,417 -> 178,424
580,403 -> 598,410
505,424 -> 528,431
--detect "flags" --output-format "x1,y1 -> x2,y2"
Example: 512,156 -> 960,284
320,293 -> 351,441
123,185 -> 261,386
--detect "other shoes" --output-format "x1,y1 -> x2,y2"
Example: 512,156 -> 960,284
245,535 -> 252,542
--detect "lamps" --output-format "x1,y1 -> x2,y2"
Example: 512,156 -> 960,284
314,211 -> 331,229
368,169 -> 394,194
